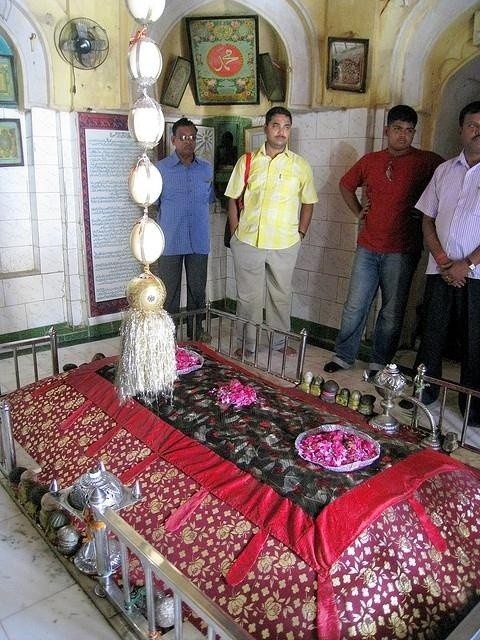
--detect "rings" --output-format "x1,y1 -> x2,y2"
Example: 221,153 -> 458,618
447,277 -> 452,283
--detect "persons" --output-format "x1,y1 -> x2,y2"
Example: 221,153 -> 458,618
223,105 -> 319,360
398,99 -> 480,428
323,103 -> 446,379
154,116 -> 217,344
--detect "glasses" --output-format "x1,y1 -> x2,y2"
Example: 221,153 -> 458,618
175,135 -> 196,141
384,158 -> 395,183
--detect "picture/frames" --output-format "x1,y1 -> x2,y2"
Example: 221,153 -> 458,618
184,15 -> 260,105
326,36 -> 369,94
243,126 -> 290,153
0,54 -> 18,105
259,52 -> 286,105
162,121 -> 216,181
0,116 -> 25,167
160,55 -> 193,108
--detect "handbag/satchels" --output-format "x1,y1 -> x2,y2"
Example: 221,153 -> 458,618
223,186 -> 247,249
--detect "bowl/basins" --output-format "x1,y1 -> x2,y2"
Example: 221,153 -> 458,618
295,424 -> 382,472
175,345 -> 204,373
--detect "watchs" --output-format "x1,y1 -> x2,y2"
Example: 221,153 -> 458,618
465,257 -> 476,271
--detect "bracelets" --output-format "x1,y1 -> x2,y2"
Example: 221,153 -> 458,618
432,251 -> 448,264
299,230 -> 307,238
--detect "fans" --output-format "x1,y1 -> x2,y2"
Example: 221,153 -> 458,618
57,18 -> 109,69
